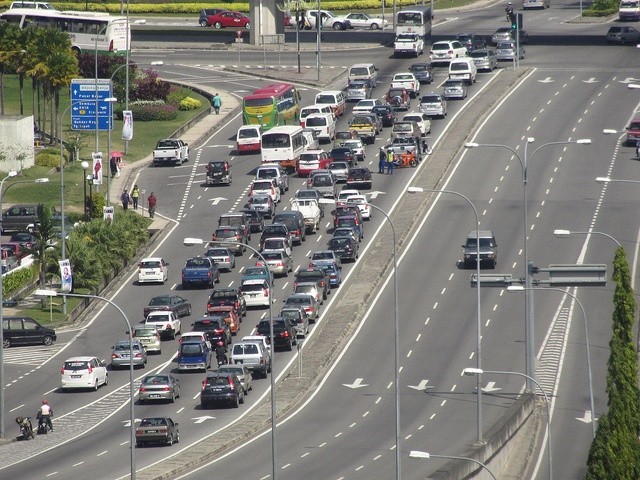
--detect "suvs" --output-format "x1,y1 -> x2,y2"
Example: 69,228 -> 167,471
429,40 -> 468,67
206,288 -> 247,323
209,226 -> 247,256
200,372 -> 245,408
461,230 -> 498,269
385,88 -> 411,112
393,32 -> 425,57
294,268 -> 331,300
255,317 -> 297,351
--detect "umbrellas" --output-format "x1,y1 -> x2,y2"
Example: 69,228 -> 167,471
111,151 -> 123,157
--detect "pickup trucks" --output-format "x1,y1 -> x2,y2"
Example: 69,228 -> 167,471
152,138 -> 190,166
289,8 -> 344,31
387,136 -> 418,158
389,72 -> 421,98
345,116 -> 376,144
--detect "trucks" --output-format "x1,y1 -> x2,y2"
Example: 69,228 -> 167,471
290,198 -> 320,234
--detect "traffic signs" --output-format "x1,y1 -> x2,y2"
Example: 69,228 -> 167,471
71,117 -> 113,131
71,79 -> 111,100
71,101 -> 113,116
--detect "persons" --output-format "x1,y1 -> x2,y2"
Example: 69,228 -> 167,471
387,149 -> 398,175
379,147 -> 387,173
110,157 -> 117,177
121,190 -> 131,210
37,399 -> 53,432
215,341 -> 227,365
212,93 -> 222,115
131,184 -> 140,210
148,192 -> 156,220
505,2 -> 513,20
115,156 -> 122,176
94,160 -> 101,179
63,268 -> 71,285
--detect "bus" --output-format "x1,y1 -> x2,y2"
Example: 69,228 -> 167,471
260,125 -> 305,174
242,83 -> 301,134
314,91 -> 346,119
394,6 -> 434,40
299,104 -> 336,130
0,8 -> 132,57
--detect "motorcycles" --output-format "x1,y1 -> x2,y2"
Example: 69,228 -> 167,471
506,11 -> 513,21
15,416 -> 35,440
216,351 -> 227,367
392,153 -> 417,168
421,140 -> 432,155
37,412 -> 50,435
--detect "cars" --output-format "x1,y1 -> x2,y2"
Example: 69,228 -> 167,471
371,104 -> 396,126
205,161 -> 232,186
143,294 -> 192,319
237,279 -> 271,309
351,99 -> 383,118
408,63 -> 433,84
144,309 -> 182,340
342,12 -> 389,30
218,364 -> 254,395
391,120 -> 423,142
254,249 -> 293,277
402,112 -> 431,137
203,306 -> 241,336
334,216 -> 363,241
455,33 -> 486,53
126,325 -> 162,354
332,129 -> 362,150
259,224 -> 290,246
308,250 -> 341,270
332,226 -> 360,245
329,236 -> 359,262
178,331 -> 212,352
333,205 -> 364,225
327,161 -> 349,182
261,237 -> 292,258
345,194 -> 372,221
345,167 -> 373,188
247,178 -> 281,205
301,128 -> 320,151
307,168 -> 332,190
336,189 -> 360,206
295,282 -> 323,306
282,295 -> 320,323
345,79 -> 371,102
138,257 -> 170,284
207,11 -> 251,31
244,195 -> 276,219
198,9 -> 225,27
191,315 -> 231,350
442,79 -> 467,100
242,335 -> 271,357
8,232 -> 38,253
60,356 -> 109,391
205,247 -> 236,273
418,94 -> 447,118
522,0 -> 550,9
494,40 -> 525,61
295,188 -> 325,219
362,112 -> 383,135
138,373 -> 181,404
111,341 -> 147,368
1,242 -> 21,272
491,27 -> 529,44
342,140 -> 366,161
469,50 -> 499,72
625,119 -> 640,145
313,263 -> 342,288
242,266 -> 275,289
135,416 -> 180,447
181,256 -> 220,288
329,146 -> 359,168
238,208 -> 265,233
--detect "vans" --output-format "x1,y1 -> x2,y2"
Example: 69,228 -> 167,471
617,0 -> 640,20
448,57 -> 478,85
312,173 -> 337,200
606,26 -> 640,44
255,165 -> 289,194
271,211 -> 306,246
278,307 -> 310,338
296,150 -> 329,177
217,212 -> 251,240
305,112 -> 336,143
0,204 -> 53,235
9,1 -> 55,11
176,341 -> 211,372
230,341 -> 271,378
2,316 -> 56,348
347,63 -> 378,88
236,124 -> 263,154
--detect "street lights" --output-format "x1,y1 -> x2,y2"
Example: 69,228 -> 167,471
32,289 -> 135,480
182,237 -> 275,480
58,97 -> 118,314
409,450 -> 496,480
0,178 -> 50,208
553,229 -> 621,247
107,61 -> 164,206
0,172 -> 18,234
86,174 -> 94,218
79,161 -> 89,214
406,186 -> 484,442
94,20 -> 147,192
507,284 -> 596,439
602,128 -> 639,142
594,176 -> 639,184
318,198 -> 401,480
463,137 -> 592,396
463,367 -> 553,480
